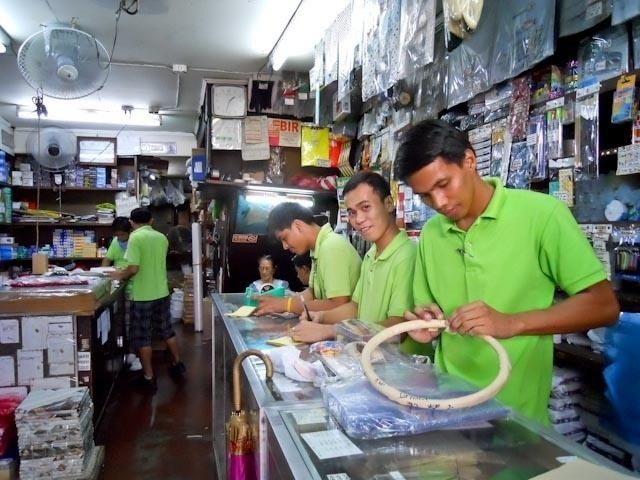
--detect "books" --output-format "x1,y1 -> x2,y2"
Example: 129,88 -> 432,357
14,386 -> 94,479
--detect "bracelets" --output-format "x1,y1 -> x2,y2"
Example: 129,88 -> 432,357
287,298 -> 292,313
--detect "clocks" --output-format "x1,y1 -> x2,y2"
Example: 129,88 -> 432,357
209,84 -> 250,119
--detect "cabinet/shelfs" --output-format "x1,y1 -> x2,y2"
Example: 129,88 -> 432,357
0,272 -> 123,429
137,156 -> 194,261
206,289 -> 639,479
0,153 -> 137,268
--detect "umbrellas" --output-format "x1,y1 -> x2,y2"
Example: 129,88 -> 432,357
226,349 -> 273,479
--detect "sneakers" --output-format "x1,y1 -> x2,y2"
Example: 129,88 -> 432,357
128,354 -> 142,370
169,361 -> 186,374
143,377 -> 157,388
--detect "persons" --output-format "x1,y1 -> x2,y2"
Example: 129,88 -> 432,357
249,254 -> 291,296
287,172 -> 431,360
103,208 -> 186,388
249,202 -> 364,317
101,217 -> 133,303
392,117 -> 620,429
291,251 -> 312,286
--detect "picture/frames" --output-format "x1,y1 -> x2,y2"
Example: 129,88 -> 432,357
75,137 -> 118,167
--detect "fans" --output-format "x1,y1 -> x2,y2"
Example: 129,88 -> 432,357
25,126 -> 79,173
16,28 -> 112,100
